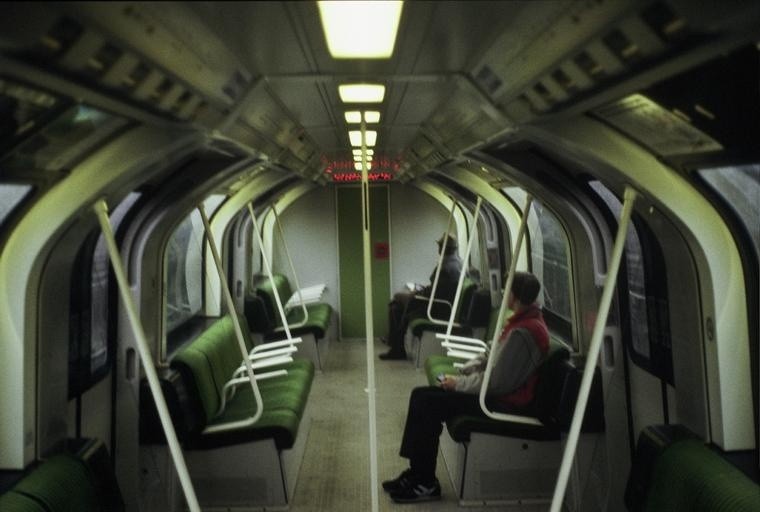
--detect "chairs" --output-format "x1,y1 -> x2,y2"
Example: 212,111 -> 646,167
623,425 -> 760,512
404,266 -> 492,370
424,307 -> 605,512
244,273 -> 332,371
138,312 -> 316,512
0,436 -> 128,512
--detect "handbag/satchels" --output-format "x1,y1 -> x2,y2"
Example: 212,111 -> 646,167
391,292 -> 416,331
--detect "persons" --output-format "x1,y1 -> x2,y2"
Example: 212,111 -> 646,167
378,232 -> 463,360
381,271 -> 550,505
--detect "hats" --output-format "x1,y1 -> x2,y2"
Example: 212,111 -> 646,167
435,231 -> 458,248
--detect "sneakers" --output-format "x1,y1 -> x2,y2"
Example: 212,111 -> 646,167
387,477 -> 442,503
382,468 -> 413,492
